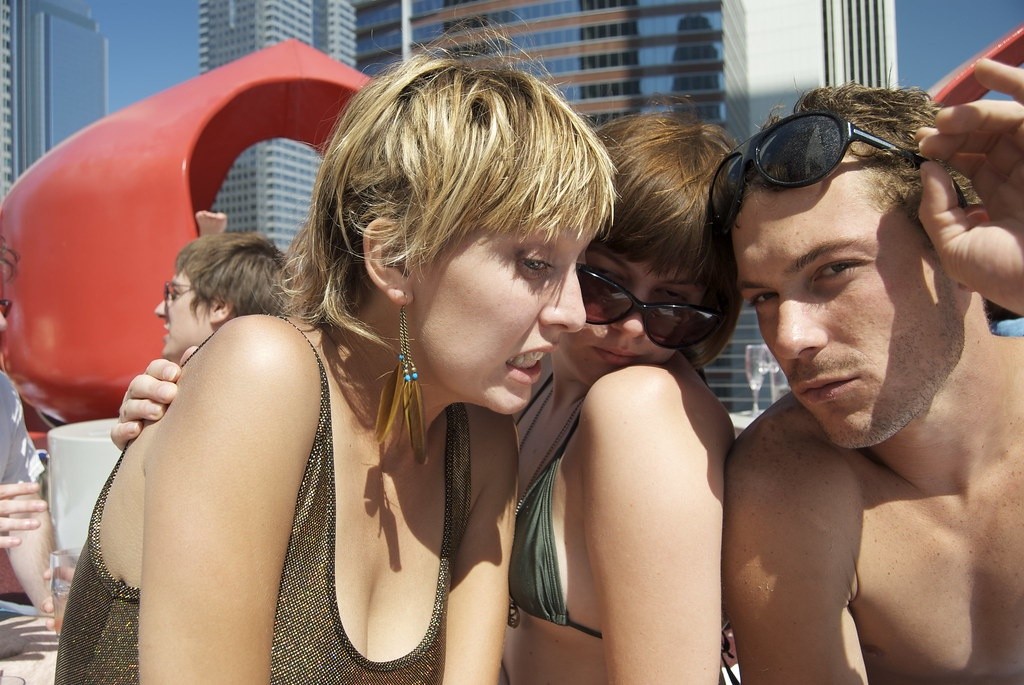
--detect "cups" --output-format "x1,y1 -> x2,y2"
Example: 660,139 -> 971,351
770,350 -> 792,406
49,547 -> 82,643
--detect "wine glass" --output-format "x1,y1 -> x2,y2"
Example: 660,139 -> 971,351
742,344 -> 768,417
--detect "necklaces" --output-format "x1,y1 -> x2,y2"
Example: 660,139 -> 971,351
508,388 -> 584,628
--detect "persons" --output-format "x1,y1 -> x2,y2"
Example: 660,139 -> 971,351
43,233 -> 292,635
0,233 -> 59,685
722,58 -> 1024,685
111,116 -> 745,685
55,53 -> 615,685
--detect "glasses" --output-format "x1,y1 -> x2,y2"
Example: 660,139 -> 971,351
166,283 -> 238,317
0,299 -> 13,318
576,264 -> 728,349
708,111 -> 969,235
